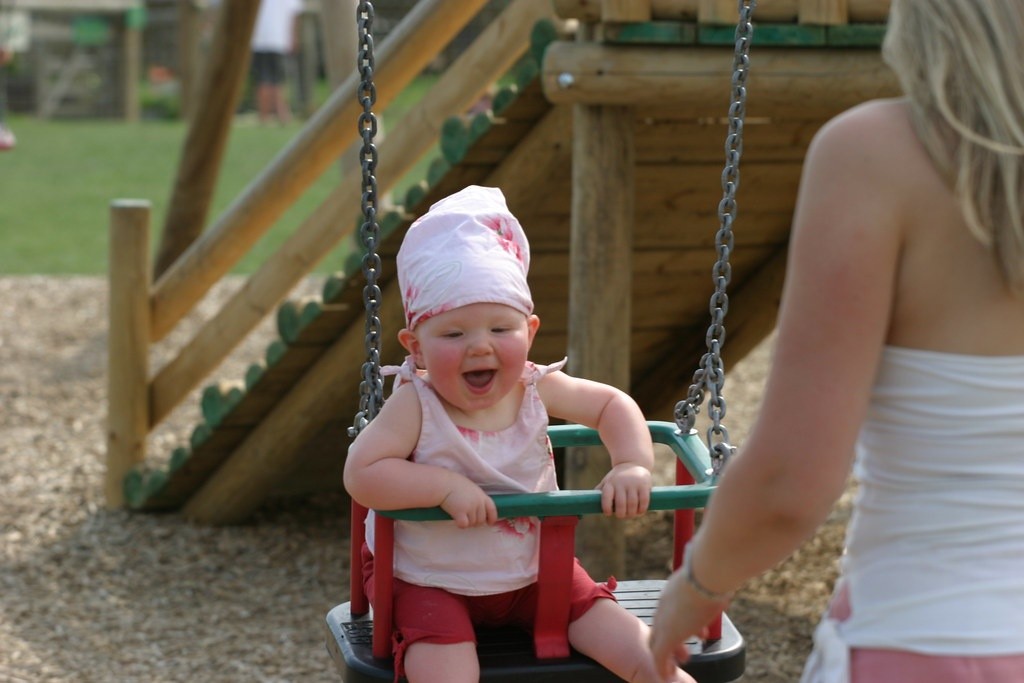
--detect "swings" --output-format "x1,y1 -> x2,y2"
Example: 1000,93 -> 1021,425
325,0 -> 752,683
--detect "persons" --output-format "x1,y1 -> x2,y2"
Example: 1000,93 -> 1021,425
342,184 -> 702,683
247,0 -> 304,125
636,0 -> 1024,683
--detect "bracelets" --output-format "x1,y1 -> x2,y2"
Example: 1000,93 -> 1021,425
683,534 -> 737,601
